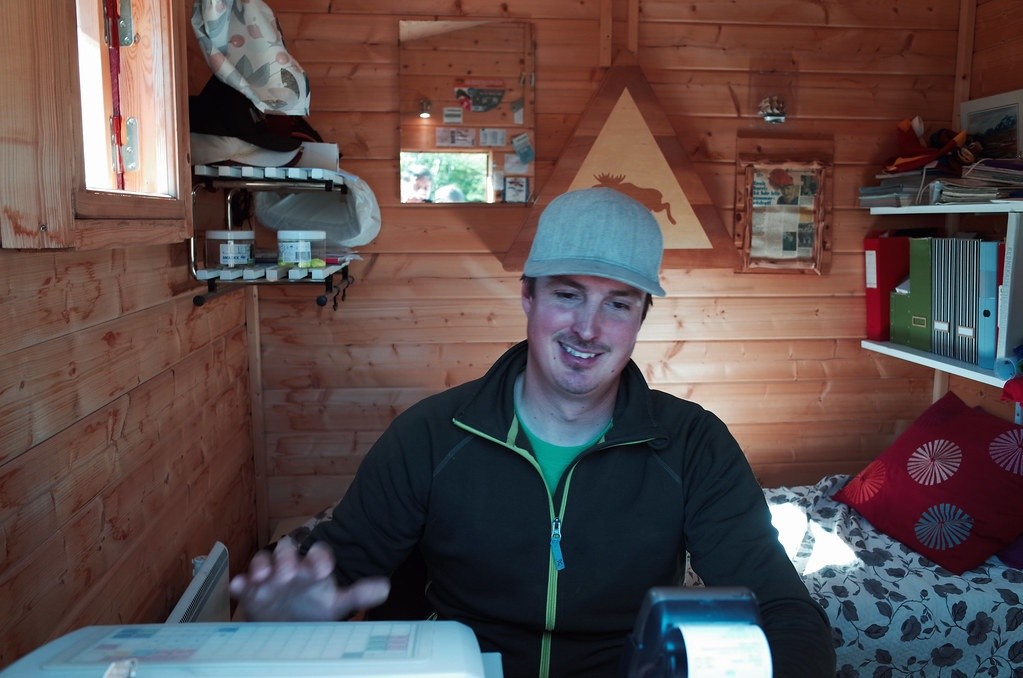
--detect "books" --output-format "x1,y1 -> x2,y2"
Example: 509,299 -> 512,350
857,156 -> 1023,208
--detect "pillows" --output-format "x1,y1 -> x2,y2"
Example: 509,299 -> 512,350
829,389 -> 1023,578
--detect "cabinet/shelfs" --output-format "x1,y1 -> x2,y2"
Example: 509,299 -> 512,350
859,200 -> 1023,402
185,162 -> 379,309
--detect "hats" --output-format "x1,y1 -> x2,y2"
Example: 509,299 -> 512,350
523,187 -> 666,297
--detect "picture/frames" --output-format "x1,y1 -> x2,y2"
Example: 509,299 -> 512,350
731,154 -> 834,276
960,89 -> 1023,159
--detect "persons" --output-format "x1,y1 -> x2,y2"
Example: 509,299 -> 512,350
777,184 -> 798,205
228,187 -> 840,678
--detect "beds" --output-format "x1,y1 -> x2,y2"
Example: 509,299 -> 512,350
230,474 -> 1023,678
